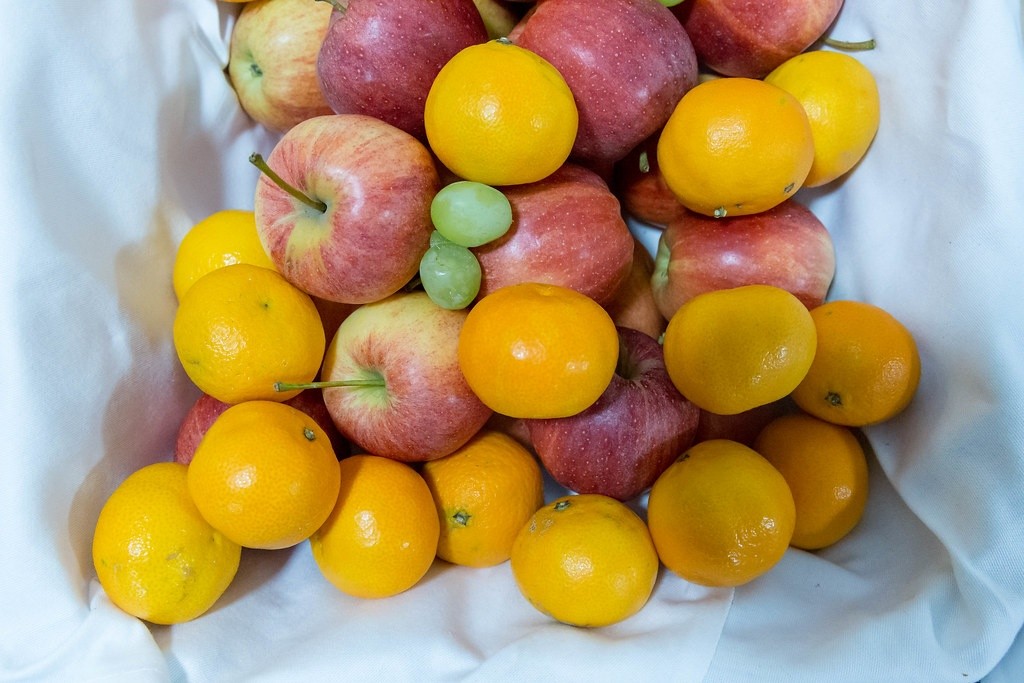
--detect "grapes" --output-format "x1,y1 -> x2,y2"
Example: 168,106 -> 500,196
430,181 -> 512,247
420,241 -> 481,310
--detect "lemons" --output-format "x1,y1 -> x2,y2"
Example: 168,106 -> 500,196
92,36 -> 920,627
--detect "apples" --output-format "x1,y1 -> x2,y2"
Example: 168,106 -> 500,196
176,0 -> 875,499
